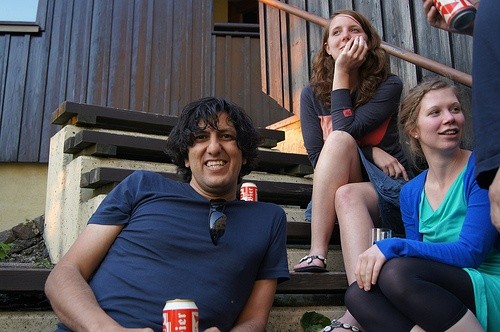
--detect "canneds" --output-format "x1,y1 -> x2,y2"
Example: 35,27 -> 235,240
432,0 -> 477,32
239,182 -> 258,202
162,299 -> 199,332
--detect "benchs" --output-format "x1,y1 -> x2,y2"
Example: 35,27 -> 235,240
0,101 -> 386,332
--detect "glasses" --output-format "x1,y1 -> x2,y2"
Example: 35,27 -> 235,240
209,199 -> 228,245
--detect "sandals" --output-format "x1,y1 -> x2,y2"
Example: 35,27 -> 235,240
294,256 -> 330,273
322,319 -> 361,332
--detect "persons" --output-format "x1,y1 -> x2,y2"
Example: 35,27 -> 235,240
423,0 -> 500,232
344,78 -> 500,332
44,97 -> 291,332
294,10 -> 412,332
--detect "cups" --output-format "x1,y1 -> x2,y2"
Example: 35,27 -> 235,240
370,228 -> 392,247
